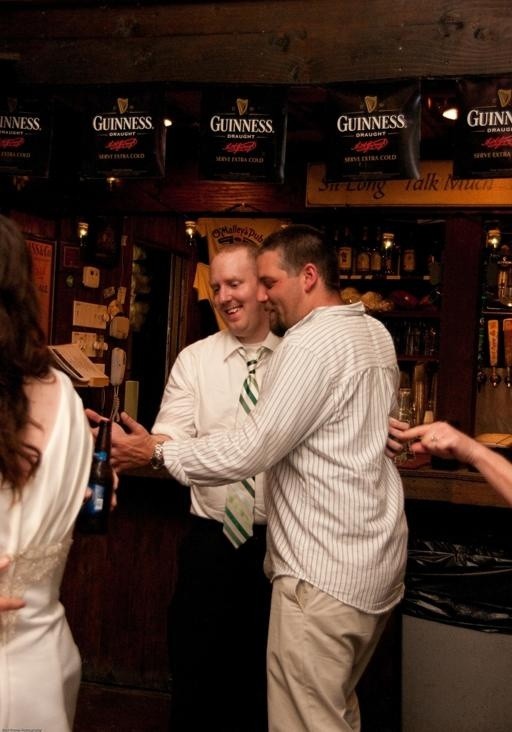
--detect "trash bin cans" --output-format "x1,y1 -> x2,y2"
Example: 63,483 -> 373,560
402,537 -> 512,732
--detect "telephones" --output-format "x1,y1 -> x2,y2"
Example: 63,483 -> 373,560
111,348 -> 127,386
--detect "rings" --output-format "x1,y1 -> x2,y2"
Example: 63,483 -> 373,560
431,437 -> 435,441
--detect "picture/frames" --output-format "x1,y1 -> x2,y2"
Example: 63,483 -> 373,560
23,234 -> 57,346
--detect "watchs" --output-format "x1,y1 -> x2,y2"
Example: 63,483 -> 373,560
150,442 -> 165,470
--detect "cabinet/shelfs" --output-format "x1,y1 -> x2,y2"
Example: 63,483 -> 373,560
187,206 -> 512,509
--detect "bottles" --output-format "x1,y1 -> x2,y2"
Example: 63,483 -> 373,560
331,227 -> 339,263
399,364 -> 411,390
414,320 -> 426,357
339,219 -> 351,275
382,233 -> 395,274
384,315 -> 395,342
393,388 -> 417,465
414,328 -> 437,425
355,222 -> 371,275
429,253 -> 441,280
372,222 -> 383,275
405,319 -> 416,357
404,249 -> 416,273
81,419 -> 113,537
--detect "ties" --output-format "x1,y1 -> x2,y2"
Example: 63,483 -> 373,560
222,347 -> 266,549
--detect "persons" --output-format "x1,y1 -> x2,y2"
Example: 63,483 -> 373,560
0,467 -> 119,611
0,215 -> 94,732
399,420 -> 512,508
